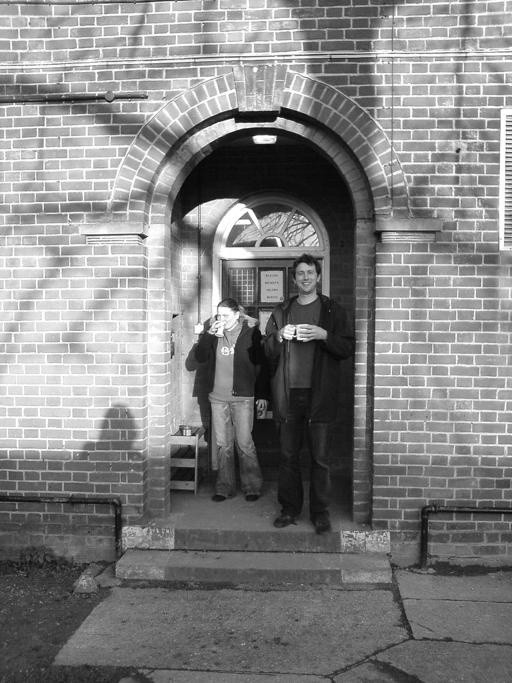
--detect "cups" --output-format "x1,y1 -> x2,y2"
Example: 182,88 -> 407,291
291,323 -> 309,341
214,322 -> 227,337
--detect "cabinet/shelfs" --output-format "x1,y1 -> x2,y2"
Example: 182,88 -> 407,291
169,420 -> 207,496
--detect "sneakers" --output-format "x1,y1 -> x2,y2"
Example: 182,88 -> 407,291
273,510 -> 301,529
211,492 -> 262,503
311,510 -> 332,533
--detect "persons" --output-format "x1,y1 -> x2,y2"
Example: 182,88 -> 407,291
195,298 -> 271,502
264,253 -> 353,534
